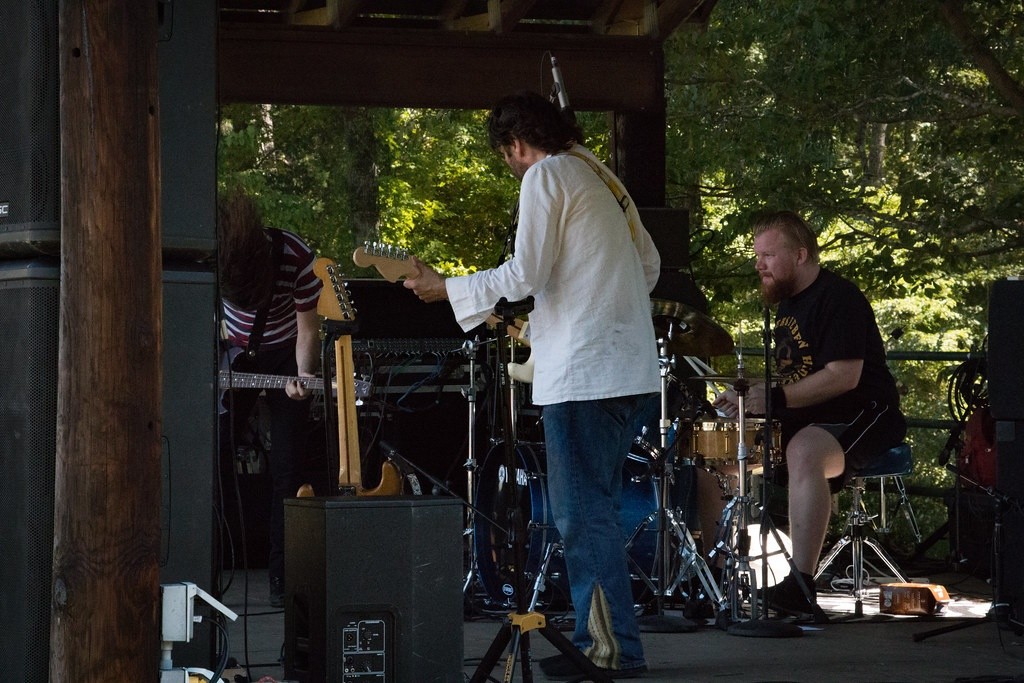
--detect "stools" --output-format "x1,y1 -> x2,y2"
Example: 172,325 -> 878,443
811,442 -> 921,618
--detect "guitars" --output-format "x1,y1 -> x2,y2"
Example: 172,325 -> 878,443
219,347 -> 372,414
297,257 -> 402,497
352,241 -> 533,383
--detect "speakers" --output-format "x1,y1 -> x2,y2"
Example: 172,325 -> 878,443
1,0 -> 216,683
284,492 -> 468,682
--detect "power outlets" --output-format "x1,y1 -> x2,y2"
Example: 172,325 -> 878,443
160,581 -> 199,644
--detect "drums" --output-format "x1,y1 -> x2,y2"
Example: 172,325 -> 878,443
472,439 -> 698,612
678,418 -> 784,465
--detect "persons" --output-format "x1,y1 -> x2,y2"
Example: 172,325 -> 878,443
403,90 -> 663,679
216,202 -> 322,608
715,210 -> 906,614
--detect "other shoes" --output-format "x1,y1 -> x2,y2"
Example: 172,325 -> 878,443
541,654 -> 649,680
749,577 -> 816,615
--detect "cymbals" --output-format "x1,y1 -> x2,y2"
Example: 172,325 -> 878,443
650,298 -> 734,356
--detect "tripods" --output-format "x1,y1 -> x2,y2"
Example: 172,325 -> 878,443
468,296 -> 614,683
912,500 -> 1024,646
